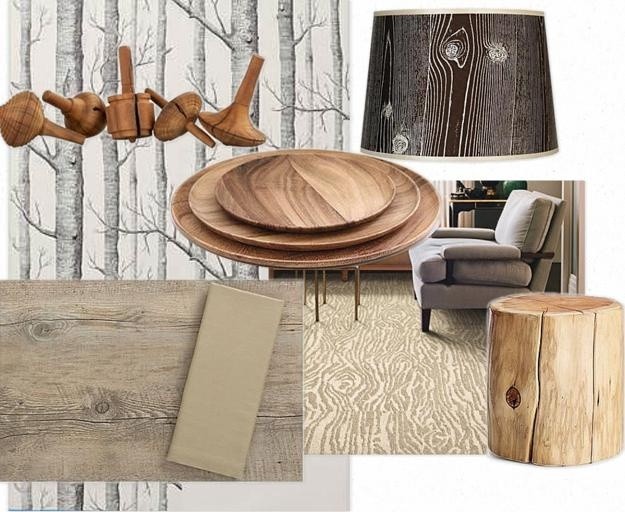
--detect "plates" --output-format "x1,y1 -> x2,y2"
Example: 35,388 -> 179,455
165,149 -> 442,274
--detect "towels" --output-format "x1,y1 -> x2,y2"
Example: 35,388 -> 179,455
458,209 -> 475,228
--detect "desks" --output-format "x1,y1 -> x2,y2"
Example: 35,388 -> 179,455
303,266 -> 361,322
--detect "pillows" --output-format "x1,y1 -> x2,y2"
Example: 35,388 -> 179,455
494,190 -> 556,264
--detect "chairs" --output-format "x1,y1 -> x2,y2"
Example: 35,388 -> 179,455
409,190 -> 568,332
